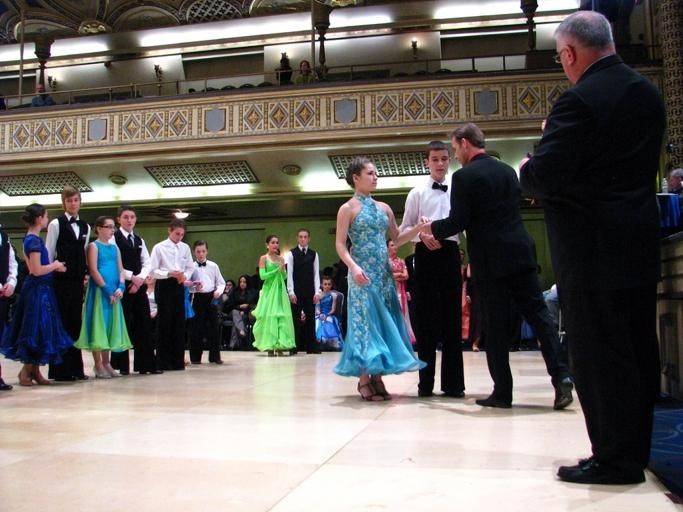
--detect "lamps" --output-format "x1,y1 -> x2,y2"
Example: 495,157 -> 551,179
410,37 -> 417,61
171,209 -> 192,220
154,63 -> 162,83
47,74 -> 56,92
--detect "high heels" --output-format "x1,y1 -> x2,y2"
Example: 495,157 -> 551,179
268,349 -> 287,357
94,364 -> 122,379
357,379 -> 392,402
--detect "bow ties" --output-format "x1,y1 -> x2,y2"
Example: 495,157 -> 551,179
198,261 -> 206,266
69,216 -> 82,226
432,182 -> 448,192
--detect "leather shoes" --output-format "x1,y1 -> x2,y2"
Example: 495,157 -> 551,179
417,387 -> 436,397
476,396 -> 513,408
17,370 -> 52,385
289,349 -> 298,355
48,369 -> 90,380
139,367 -> 164,374
445,387 -> 465,399
120,368 -> 130,375
191,360 -> 201,364
556,455 -> 647,486
209,357 -> 223,364
552,376 -> 574,410
306,349 -> 321,354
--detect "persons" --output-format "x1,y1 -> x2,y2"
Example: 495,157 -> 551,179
399,141 -> 467,398
286,228 -> 322,355
419,123 -> 573,410
315,275 -> 343,350
32,84 -> 55,106
386,239 -> 417,345
250,234 -> 297,356
667,167 -> 683,194
404,243 -> 417,351
518,9 -> 667,486
464,263 -> 481,352
519,284 -> 561,350
295,61 -> 320,86
459,249 -> 470,350
335,156 -> 431,402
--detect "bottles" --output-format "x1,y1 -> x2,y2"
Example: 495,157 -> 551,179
661,176 -> 669,193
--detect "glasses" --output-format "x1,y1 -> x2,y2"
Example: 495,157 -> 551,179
554,49 -> 564,64
101,224 -> 116,230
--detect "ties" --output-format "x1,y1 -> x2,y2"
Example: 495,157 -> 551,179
128,234 -> 134,247
302,247 -> 305,255
